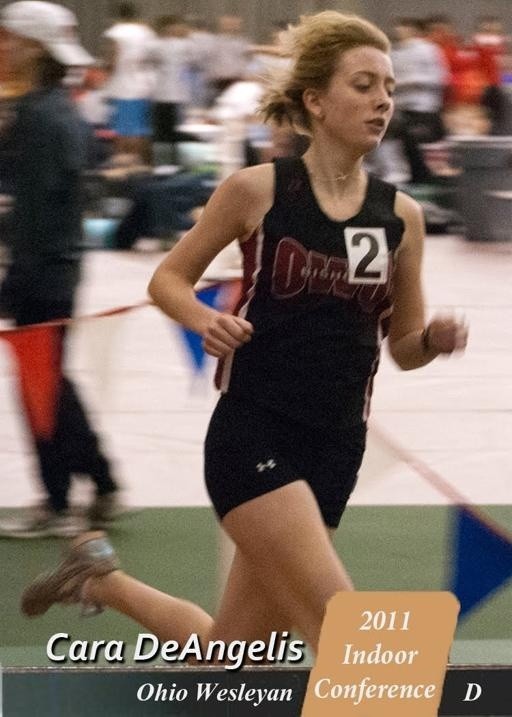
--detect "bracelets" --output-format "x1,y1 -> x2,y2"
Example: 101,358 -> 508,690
409,321 -> 431,366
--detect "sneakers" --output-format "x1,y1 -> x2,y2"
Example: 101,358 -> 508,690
84,492 -> 127,525
20,529 -> 123,617
0,509 -> 82,541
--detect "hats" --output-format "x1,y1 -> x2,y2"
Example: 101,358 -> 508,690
1,1 -> 98,67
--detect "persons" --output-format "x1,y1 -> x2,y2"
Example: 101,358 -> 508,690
17,10 -> 479,671
1,0 -> 145,540
71,0 -> 512,255
